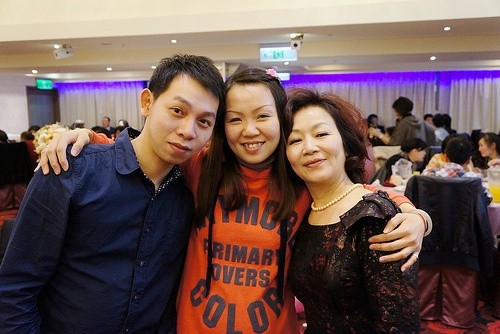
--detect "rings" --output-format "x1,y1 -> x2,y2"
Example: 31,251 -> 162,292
413,253 -> 418,259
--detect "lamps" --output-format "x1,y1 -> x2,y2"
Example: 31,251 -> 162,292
53,44 -> 76,59
291,32 -> 304,50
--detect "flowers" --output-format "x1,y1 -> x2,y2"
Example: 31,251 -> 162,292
33,122 -> 70,162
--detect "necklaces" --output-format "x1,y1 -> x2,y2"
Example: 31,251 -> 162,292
311,183 -> 364,212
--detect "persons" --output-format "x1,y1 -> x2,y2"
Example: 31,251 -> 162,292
102,116 -> 114,134
424,113 -> 457,134
367,114 -> 386,146
475,132 -> 500,173
92,126 -> 112,139
422,133 -> 492,205
71,120 -> 84,129
113,120 -> 129,141
384,137 -> 427,182
35,68 -> 432,334
367,96 -> 431,173
20,125 -> 40,169
280,84 -> 424,334
0,130 -> 8,144
0,54 -> 224,334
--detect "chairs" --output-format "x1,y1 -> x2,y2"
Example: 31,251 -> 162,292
402,177 -> 495,327
0,141 -> 34,210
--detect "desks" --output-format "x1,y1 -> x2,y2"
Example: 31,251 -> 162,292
392,174 -> 500,231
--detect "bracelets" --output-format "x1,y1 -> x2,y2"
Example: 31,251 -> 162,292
408,209 -> 432,237
379,134 -> 384,139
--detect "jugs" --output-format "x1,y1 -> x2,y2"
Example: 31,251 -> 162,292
488,171 -> 500,203
